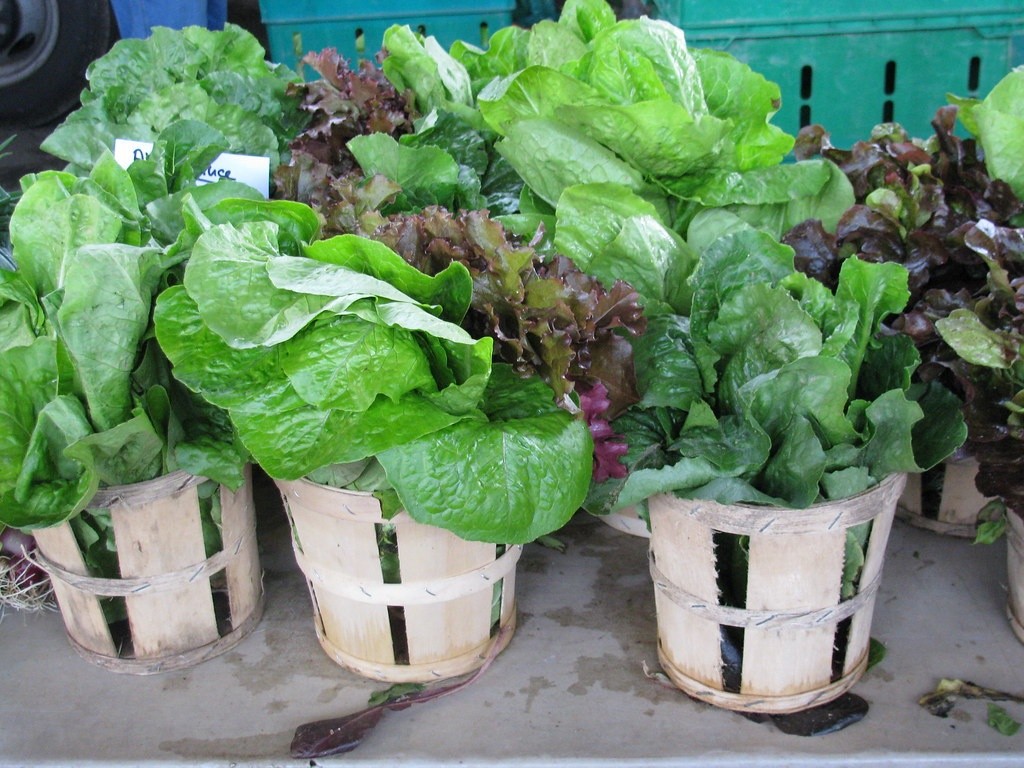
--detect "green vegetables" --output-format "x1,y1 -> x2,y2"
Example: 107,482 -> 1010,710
0,0 -> 1023,673
